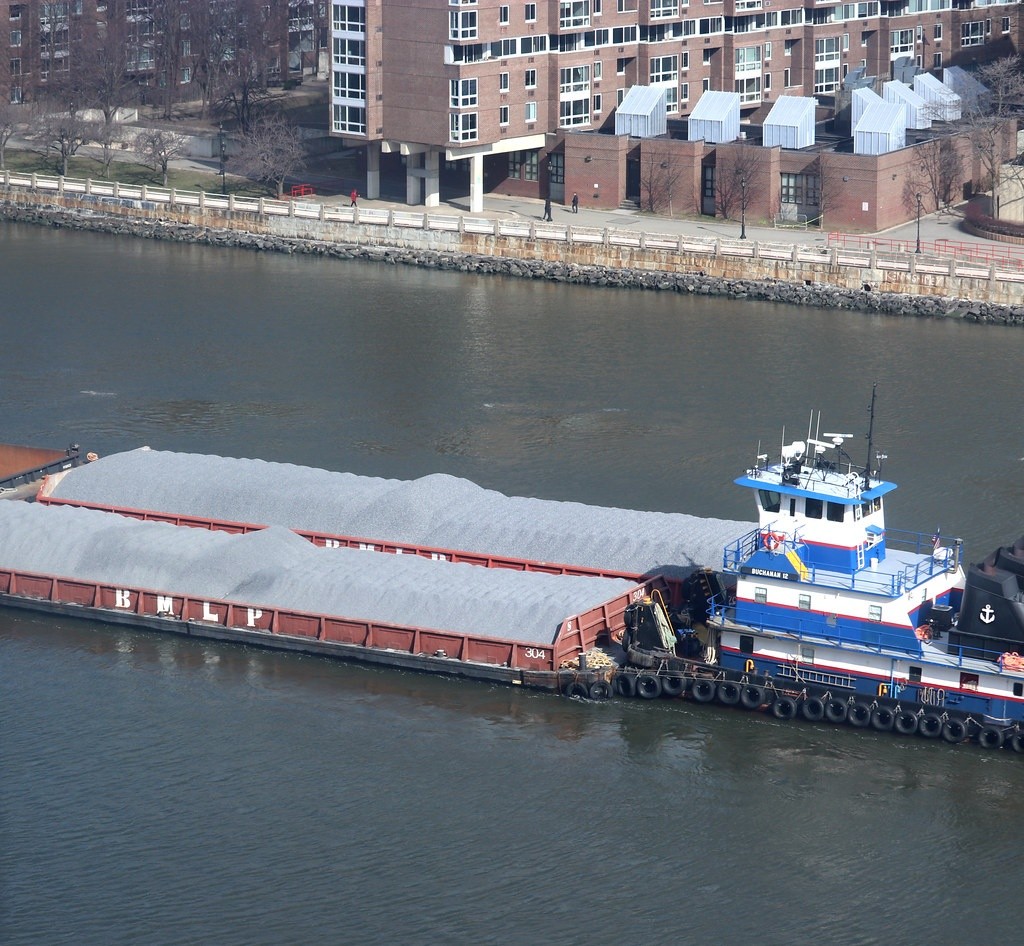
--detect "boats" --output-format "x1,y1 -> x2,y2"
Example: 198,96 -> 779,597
621,381 -> 1024,754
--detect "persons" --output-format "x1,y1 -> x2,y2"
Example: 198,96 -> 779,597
571,192 -> 578,213
350,189 -> 357,207
543,197 -> 550,219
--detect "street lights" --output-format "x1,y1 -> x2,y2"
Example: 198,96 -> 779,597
741,176 -> 748,239
546,161 -> 553,221
216,121 -> 224,175
915,192 -> 922,254
221,141 -> 227,198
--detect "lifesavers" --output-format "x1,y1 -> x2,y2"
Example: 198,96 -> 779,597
565,672 -> 1024,754
622,604 -> 637,652
764,533 -> 779,550
